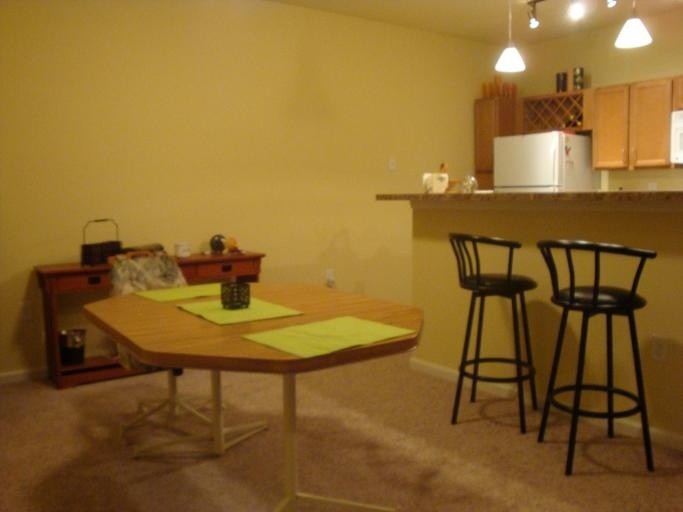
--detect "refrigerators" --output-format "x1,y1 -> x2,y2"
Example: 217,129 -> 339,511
491,132 -> 600,191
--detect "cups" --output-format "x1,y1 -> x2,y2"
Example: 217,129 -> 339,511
96,334 -> 121,361
483,75 -> 515,99
59,329 -> 85,366
173,243 -> 191,258
555,72 -> 566,93
572,68 -> 583,90
221,282 -> 249,310
422,173 -> 447,194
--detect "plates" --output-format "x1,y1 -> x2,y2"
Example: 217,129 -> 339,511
445,179 -> 458,192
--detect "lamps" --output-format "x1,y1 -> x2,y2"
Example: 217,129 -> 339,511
494,0 -> 654,75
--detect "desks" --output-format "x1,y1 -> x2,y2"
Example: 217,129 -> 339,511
84,282 -> 426,511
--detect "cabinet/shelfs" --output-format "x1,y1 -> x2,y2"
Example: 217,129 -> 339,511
31,250 -> 266,389
592,76 -> 682,171
473,96 -> 512,175
516,88 -> 590,132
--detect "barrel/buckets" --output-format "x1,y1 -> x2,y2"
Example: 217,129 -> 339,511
59,327 -> 88,363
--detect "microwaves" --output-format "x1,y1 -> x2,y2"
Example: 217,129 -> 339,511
670,109 -> 683,164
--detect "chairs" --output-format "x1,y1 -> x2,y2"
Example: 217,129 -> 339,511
533,236 -> 658,476
444,230 -> 537,434
